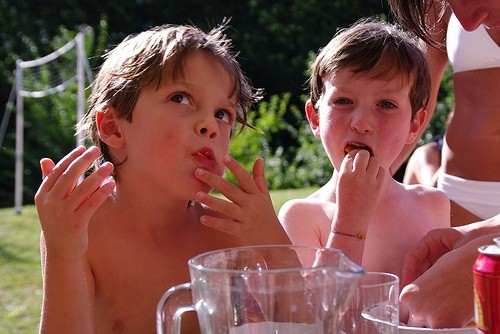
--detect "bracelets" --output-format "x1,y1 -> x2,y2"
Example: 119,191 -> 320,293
332,231 -> 367,239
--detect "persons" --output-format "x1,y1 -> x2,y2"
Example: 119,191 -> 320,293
278,18 -> 451,334
403,135 -> 443,187
402,0 -> 500,226
35,23 -> 315,334
388,0 -> 499,329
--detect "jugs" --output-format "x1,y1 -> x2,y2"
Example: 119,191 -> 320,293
156,245 -> 364,334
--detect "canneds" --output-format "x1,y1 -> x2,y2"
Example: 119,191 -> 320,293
472,237 -> 500,334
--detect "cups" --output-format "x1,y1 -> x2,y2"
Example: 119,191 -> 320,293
341,271 -> 399,334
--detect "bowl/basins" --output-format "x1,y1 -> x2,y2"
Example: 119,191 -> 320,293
361,301 -> 478,334
